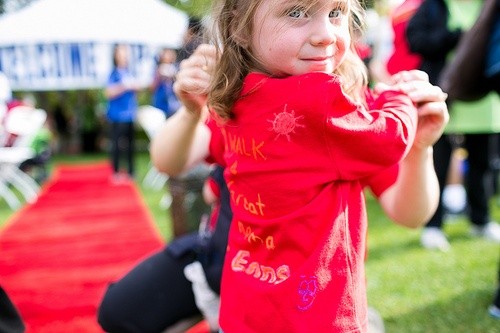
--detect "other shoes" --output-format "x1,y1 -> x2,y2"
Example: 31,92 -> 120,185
419,225 -> 450,250
473,219 -> 500,241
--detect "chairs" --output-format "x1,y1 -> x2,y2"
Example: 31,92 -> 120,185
0,106 -> 47,210
168,177 -> 204,238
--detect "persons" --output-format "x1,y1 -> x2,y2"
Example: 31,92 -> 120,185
97,43 -> 449,333
102,13 -> 213,182
205,0 -> 419,333
349,0 -> 500,323
